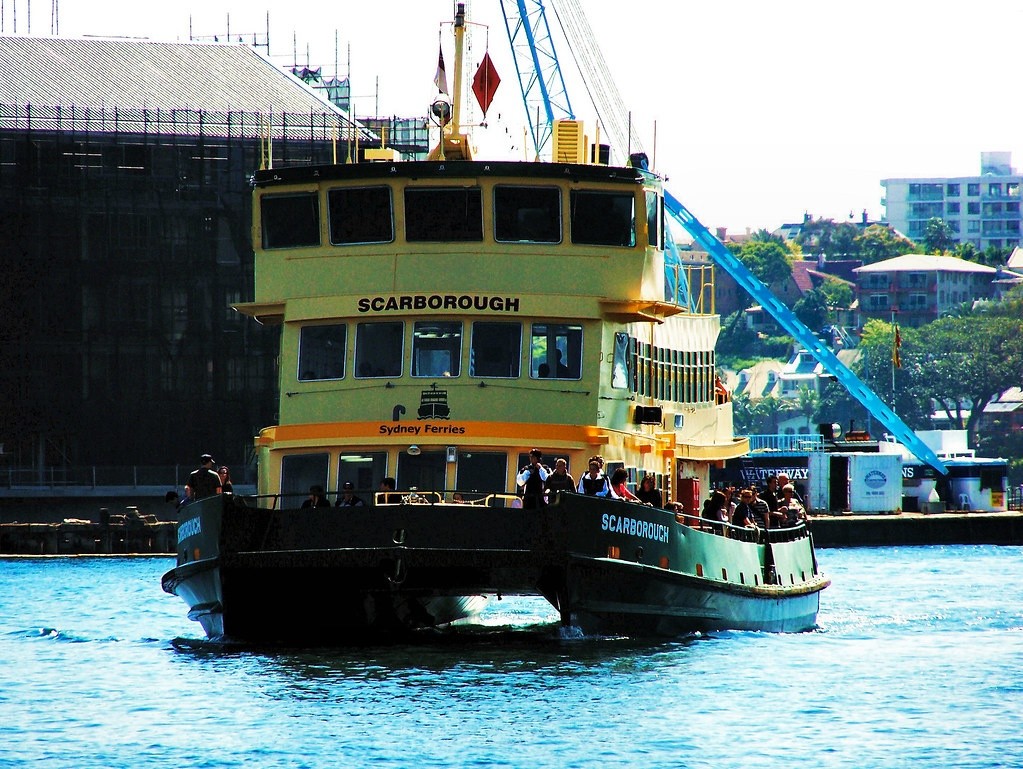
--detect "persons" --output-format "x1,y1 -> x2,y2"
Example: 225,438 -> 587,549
335,482 -> 363,508
452,492 -> 465,502
664,501 -> 685,524
301,485 -> 333,509
538,349 -> 570,379
702,473 -> 808,539
515,448 -> 662,510
377,478 -> 401,504
164,453 -> 234,560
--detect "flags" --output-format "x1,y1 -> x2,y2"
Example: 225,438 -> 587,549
894,323 -> 901,369
472,51 -> 501,120
433,43 -> 450,95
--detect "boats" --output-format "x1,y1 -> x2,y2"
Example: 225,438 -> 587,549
156,114 -> 831,640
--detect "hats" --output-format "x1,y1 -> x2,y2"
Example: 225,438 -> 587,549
342,480 -> 354,488
200,454 -> 215,464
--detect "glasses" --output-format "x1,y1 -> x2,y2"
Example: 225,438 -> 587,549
343,486 -> 351,490
220,471 -> 227,474
743,496 -> 752,498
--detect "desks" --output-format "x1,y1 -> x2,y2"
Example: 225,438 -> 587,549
0,521 -> 179,554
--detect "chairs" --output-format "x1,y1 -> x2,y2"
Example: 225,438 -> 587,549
959,494 -> 971,511
